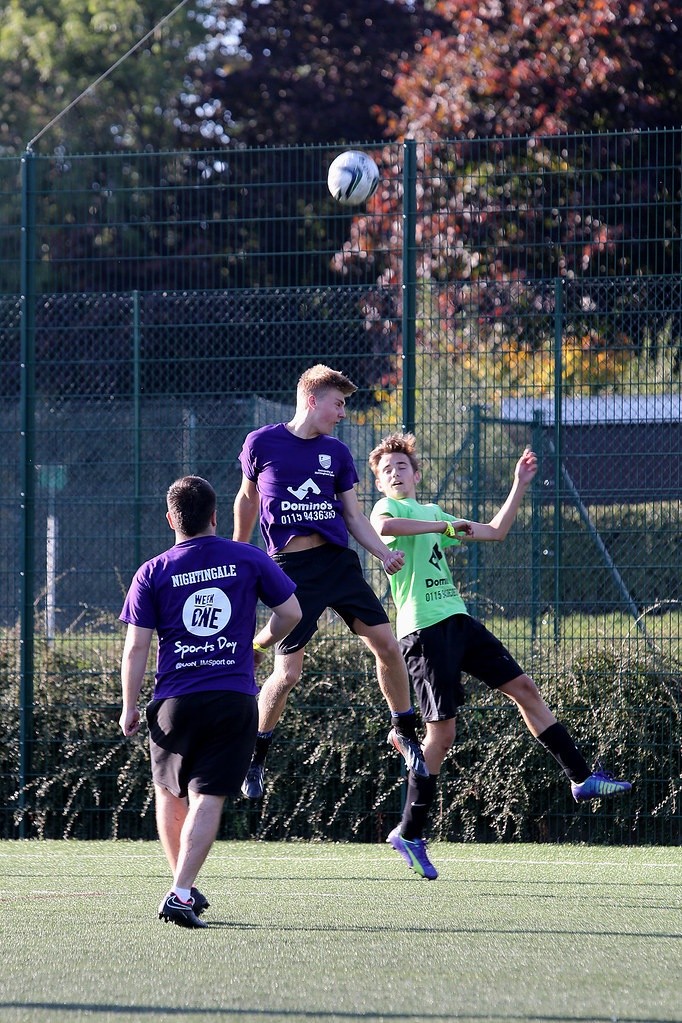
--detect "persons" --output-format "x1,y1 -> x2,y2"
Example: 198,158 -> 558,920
371,438 -> 633,881
119,476 -> 302,928
233,364 -> 427,800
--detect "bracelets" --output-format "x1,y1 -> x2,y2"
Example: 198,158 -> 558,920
443,521 -> 455,538
253,641 -> 266,654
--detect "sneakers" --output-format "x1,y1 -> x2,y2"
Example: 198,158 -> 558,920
185,887 -> 208,917
570,767 -> 633,804
387,729 -> 430,778
240,756 -> 266,799
386,825 -> 439,880
157,892 -> 209,930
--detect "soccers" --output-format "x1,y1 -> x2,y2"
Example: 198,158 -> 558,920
327,150 -> 382,206
